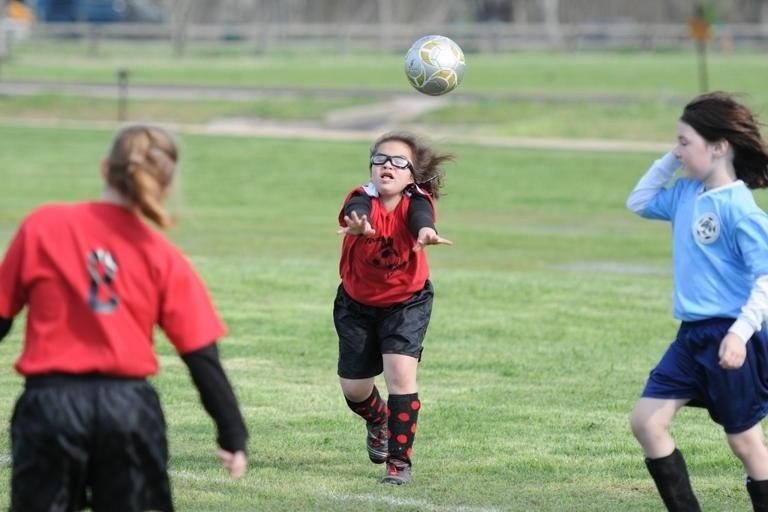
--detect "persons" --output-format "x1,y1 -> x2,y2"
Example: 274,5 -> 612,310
333,134 -> 456,485
0,125 -> 248,512
625,93 -> 768,510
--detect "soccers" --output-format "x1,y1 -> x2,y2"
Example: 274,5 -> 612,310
404,34 -> 467,94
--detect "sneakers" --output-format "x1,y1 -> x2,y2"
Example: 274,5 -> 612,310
366,420 -> 389,465
380,458 -> 411,484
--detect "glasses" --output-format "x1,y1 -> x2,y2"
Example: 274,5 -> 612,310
369,153 -> 416,180
